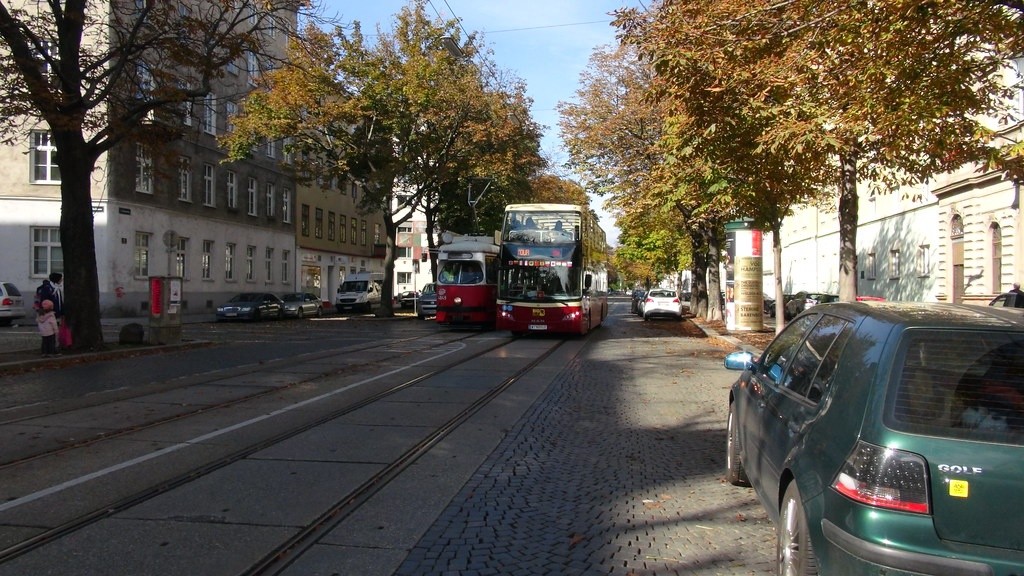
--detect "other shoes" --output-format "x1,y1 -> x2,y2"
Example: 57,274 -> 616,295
47,354 -> 52,357
41,354 -> 46,358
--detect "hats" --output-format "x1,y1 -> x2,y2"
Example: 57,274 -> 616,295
49,273 -> 62,283
42,300 -> 53,309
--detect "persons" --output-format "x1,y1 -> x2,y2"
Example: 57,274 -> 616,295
551,220 -> 562,231
1003,282 -> 1024,308
37,300 -> 59,357
525,217 -> 537,229
36,272 -> 63,323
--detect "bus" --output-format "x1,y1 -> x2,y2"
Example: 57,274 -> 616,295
432,229 -> 533,332
493,203 -> 611,337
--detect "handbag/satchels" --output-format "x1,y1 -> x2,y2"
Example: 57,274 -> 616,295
58,317 -> 72,346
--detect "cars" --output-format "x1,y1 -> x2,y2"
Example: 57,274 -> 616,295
214,291 -> 285,323
416,283 -> 438,320
399,290 -> 420,309
763,290 -> 840,323
721,297 -> 1024,576
630,287 -> 691,322
279,291 -> 323,318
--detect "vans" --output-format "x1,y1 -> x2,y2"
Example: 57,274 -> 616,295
336,272 -> 385,316
0,282 -> 27,327
